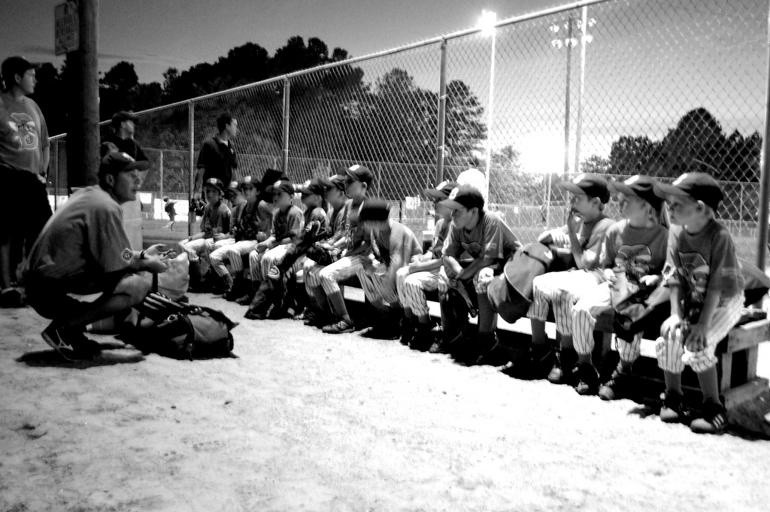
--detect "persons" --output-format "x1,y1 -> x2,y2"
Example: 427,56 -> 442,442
0,55 -> 177,362
179,112 -> 524,362
508,170 -> 745,432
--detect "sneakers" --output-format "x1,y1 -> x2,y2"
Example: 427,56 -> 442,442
510,335 -> 686,424
689,393 -> 729,434
40,318 -> 102,366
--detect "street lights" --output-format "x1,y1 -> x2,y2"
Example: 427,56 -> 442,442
477,9 -> 497,210
549,14 -> 596,228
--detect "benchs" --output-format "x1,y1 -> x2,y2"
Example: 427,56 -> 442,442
344,275 -> 770,395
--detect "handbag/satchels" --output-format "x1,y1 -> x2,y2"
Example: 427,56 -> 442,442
112,288 -> 240,361
485,240 -> 556,324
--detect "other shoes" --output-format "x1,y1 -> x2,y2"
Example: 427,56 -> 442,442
190,269 -> 499,365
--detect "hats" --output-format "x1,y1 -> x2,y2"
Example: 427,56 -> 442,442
607,173 -> 665,213
110,111 -> 144,129
652,169 -> 724,214
199,164 -> 392,222
424,179 -> 485,212
96,150 -> 151,175
1,56 -> 41,80
561,173 -> 611,205
